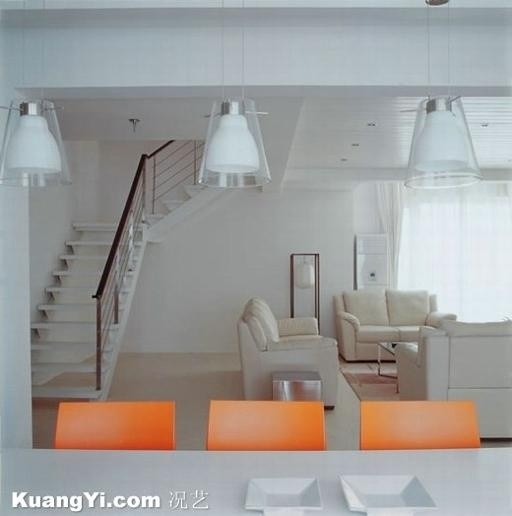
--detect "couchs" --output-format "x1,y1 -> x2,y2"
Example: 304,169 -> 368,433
238,296 -> 337,410
334,290 -> 457,362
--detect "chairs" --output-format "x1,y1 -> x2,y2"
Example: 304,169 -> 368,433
395,319 -> 511,438
50,400 -> 482,449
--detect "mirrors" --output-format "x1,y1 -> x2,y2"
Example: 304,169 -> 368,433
291,254 -> 318,318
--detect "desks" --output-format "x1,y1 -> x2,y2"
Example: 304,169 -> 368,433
2,448 -> 510,515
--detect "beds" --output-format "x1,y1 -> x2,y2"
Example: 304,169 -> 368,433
271,372 -> 322,401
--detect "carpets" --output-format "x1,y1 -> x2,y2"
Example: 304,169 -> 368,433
340,370 -> 399,400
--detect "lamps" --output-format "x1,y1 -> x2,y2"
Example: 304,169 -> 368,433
199,1 -> 271,190
405,0 -> 484,189
296,255 -> 315,289
0,1 -> 60,189
